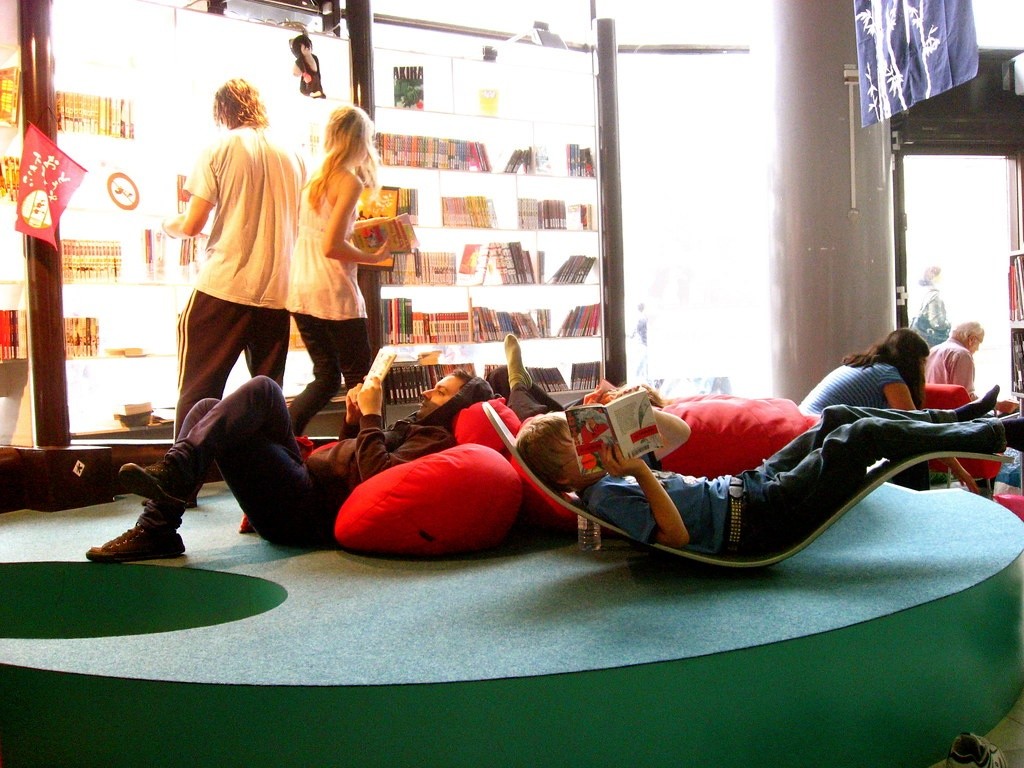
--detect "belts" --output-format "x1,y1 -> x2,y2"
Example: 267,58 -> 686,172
726,476 -> 743,548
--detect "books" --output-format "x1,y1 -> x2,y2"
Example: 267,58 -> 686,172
362,347 -> 396,391
284,134 -> 603,411
1008,254 -> 1024,394
0,67 -> 215,360
565,389 -> 663,477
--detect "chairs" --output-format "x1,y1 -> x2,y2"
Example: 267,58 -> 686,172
923,382 -> 1004,494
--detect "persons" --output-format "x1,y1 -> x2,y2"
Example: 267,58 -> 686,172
914,267 -> 950,347
486,333 -> 663,420
799,328 -> 930,418
920,321 -> 1021,419
285,105 -> 392,438
85,367 -> 493,564
143,78 -> 305,508
517,384 -> 1024,560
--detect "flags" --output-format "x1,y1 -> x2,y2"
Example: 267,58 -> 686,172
853,0 -> 979,130
13,125 -> 87,251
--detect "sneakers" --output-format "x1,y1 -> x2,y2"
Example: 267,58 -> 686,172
86,522 -> 185,562
117,458 -> 199,508
947,731 -> 1008,768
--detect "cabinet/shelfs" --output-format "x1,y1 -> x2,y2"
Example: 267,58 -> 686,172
18,0 -> 629,446
1008,249 -> 1024,398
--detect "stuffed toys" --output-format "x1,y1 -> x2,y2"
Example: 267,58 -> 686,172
288,36 -> 326,99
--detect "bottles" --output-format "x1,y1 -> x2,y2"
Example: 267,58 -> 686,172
576,514 -> 602,552
